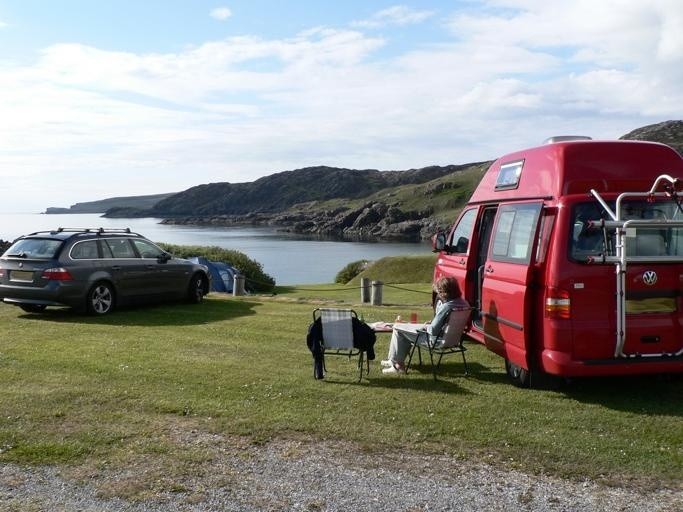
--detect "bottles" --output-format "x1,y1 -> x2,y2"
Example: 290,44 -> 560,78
358,312 -> 364,323
394,316 -> 401,324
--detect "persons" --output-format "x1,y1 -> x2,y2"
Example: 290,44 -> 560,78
378,275 -> 467,376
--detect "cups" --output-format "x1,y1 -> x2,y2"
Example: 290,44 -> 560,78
410,313 -> 416,323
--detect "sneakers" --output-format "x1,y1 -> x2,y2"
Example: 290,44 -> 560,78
380,359 -> 396,367
381,364 -> 405,374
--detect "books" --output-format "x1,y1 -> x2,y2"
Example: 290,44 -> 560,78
391,319 -> 431,336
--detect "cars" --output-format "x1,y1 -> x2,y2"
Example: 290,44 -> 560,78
0,228 -> 212,316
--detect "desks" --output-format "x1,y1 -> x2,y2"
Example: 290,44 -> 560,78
364,321 -> 421,375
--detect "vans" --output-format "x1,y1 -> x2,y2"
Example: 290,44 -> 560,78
431,136 -> 682,388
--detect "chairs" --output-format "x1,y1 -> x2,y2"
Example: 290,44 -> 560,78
390,306 -> 472,380
306,308 -> 376,387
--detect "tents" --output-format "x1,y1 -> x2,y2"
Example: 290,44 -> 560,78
189,256 -> 243,293
